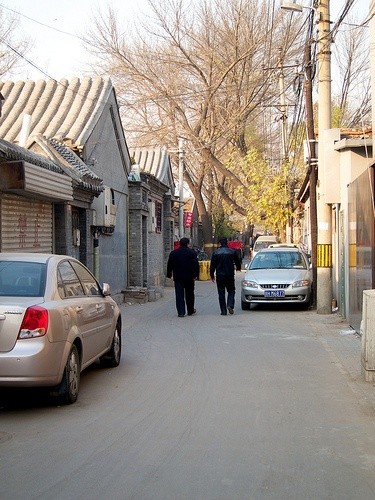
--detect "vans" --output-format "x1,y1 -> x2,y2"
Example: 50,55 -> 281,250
249,235 -> 280,261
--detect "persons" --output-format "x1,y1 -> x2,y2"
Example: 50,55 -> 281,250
231,243 -> 254,265
167,238 -> 200,317
210,237 -> 241,315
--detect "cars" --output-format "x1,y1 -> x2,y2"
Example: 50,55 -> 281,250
1,253 -> 123,406
269,244 -> 300,250
241,247 -> 312,310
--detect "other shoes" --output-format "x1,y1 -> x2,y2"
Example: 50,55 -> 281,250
221,312 -> 227,315
178,314 -> 185,317
228,306 -> 234,314
188,309 -> 196,316
